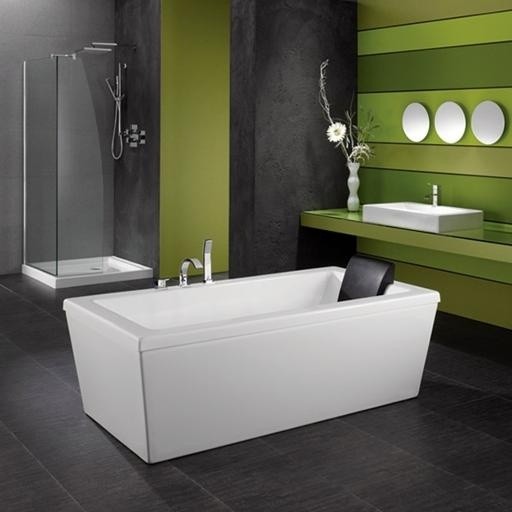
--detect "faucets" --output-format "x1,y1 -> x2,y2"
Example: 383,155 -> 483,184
424,184 -> 438,206
179,257 -> 203,284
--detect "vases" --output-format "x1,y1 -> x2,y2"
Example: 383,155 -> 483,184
345,162 -> 361,213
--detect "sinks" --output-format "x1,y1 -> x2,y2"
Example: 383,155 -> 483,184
362,202 -> 483,235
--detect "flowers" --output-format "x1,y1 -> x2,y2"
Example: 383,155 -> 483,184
316,58 -> 381,163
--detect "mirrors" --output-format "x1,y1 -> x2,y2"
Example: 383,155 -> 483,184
400,97 -> 508,147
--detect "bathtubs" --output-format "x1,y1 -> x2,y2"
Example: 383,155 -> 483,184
62,265 -> 440,465
